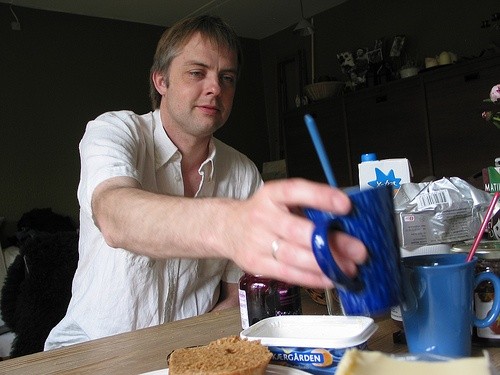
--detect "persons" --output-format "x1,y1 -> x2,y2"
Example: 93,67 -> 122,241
44,9 -> 367,353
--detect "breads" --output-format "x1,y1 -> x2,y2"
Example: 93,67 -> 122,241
169,335 -> 273,375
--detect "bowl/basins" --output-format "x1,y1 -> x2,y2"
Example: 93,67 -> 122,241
239,314 -> 379,374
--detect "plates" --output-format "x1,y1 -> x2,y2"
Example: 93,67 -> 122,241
140,364 -> 314,375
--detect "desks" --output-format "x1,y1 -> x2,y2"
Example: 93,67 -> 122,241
0,295 -> 500,375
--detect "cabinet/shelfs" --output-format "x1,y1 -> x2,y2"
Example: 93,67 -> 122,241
279,49 -> 500,188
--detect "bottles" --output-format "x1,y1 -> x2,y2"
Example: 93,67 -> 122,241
237,269 -> 304,330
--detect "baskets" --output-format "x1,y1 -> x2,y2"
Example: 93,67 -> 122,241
303,81 -> 345,101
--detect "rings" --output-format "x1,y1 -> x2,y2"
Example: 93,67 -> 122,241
270,237 -> 283,260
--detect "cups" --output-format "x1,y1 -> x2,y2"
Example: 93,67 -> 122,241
452,240 -> 500,346
304,185 -> 405,315
399,253 -> 500,361
